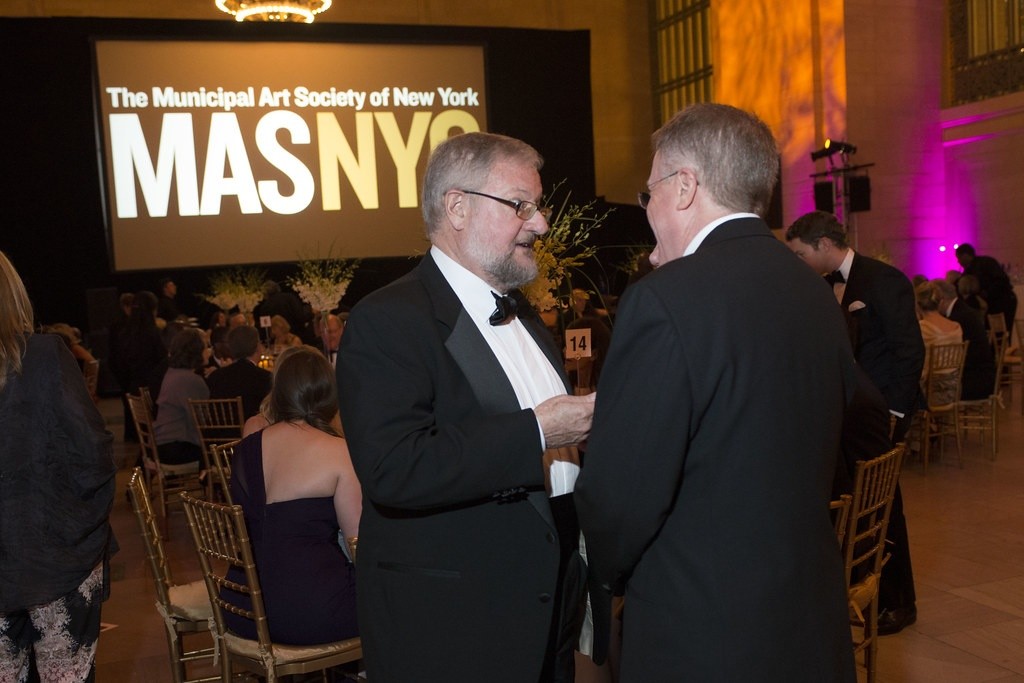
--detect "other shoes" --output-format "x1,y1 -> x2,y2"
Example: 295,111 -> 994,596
877,607 -> 917,636
850,599 -> 887,627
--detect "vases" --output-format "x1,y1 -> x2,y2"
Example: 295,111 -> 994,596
318,313 -> 331,366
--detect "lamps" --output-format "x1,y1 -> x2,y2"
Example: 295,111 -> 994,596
214,0 -> 334,23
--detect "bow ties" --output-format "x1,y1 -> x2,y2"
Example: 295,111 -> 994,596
824,271 -> 846,289
489,289 -> 529,326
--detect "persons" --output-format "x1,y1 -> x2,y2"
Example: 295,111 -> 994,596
111,280 -> 342,504
0,251 -> 115,683
572,103 -> 857,683
337,133 -> 596,683
787,211 -> 924,633
914,243 -> 1017,442
37,324 -> 95,373
558,289 -> 612,396
220,352 -> 362,646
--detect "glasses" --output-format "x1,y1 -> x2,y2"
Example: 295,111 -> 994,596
443,189 -> 538,221
638,171 -> 699,209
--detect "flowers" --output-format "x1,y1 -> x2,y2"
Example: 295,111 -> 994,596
514,176 -> 615,333
284,234 -> 359,331
191,262 -> 271,327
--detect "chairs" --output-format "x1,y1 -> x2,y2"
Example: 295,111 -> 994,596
126,465 -> 262,683
178,490 -> 367,683
828,311 -> 1023,683
81,358 -> 248,542
